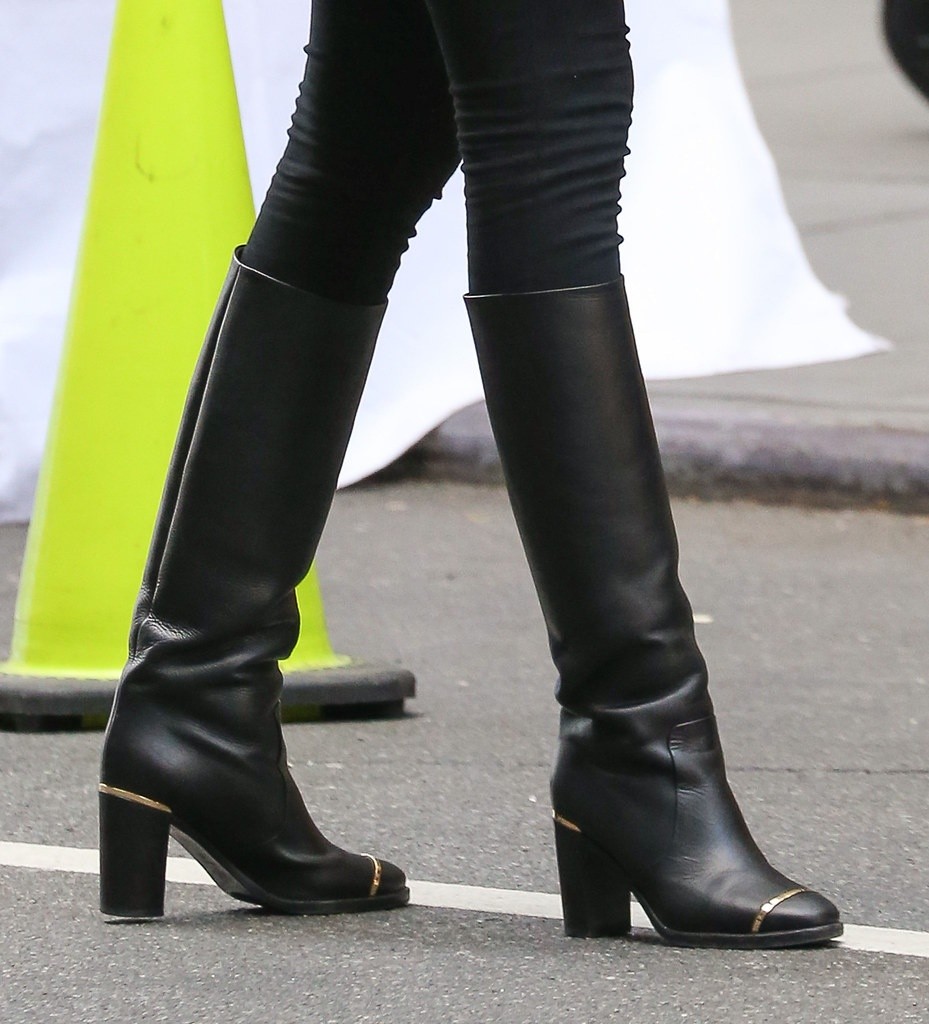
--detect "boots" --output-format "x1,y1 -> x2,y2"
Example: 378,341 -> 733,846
459,276 -> 848,949
97,242 -> 412,922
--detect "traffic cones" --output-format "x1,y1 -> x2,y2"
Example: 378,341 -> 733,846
1,0 -> 418,731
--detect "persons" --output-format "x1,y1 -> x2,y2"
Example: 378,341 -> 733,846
97,0 -> 843,950
